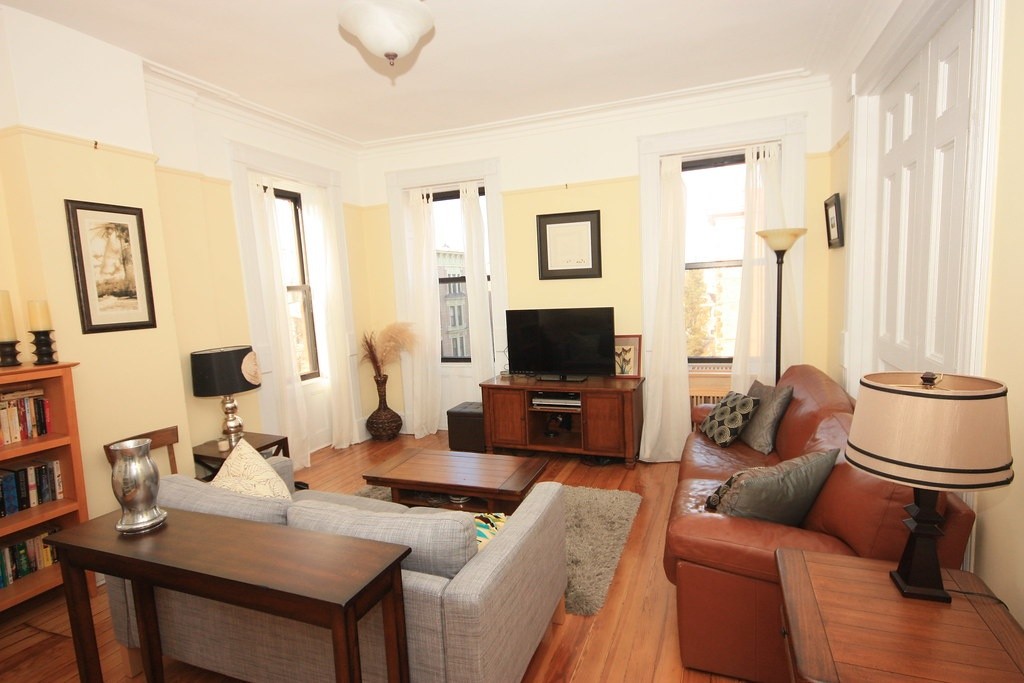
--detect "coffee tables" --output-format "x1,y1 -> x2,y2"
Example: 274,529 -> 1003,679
362,447 -> 551,515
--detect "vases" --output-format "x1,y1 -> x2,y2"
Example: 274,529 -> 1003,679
366,374 -> 403,441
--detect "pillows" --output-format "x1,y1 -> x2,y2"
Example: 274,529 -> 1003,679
211,438 -> 292,501
473,512 -> 505,551
699,379 -> 841,529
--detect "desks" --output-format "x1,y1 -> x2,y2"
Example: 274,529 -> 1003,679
42,506 -> 412,683
192,432 -> 289,477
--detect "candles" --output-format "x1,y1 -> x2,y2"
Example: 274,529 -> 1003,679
29,299 -> 51,331
0,290 -> 16,341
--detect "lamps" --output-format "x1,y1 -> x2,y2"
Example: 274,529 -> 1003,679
190,345 -> 263,448
756,228 -> 807,386
843,372 -> 1014,603
340,0 -> 434,67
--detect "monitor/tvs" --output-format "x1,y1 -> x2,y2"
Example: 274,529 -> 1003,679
506,307 -> 617,381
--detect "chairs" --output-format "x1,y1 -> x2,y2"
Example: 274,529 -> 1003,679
104,425 -> 180,474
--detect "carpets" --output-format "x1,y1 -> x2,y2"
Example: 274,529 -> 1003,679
353,481 -> 643,614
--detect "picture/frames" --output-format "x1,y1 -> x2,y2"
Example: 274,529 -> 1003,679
536,209 -> 602,280
64,199 -> 157,334
824,193 -> 844,248
608,335 -> 642,378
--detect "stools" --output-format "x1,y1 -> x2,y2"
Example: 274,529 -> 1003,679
447,402 -> 486,453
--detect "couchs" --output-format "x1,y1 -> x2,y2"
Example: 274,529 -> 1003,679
663,366 -> 976,683
105,456 -> 568,683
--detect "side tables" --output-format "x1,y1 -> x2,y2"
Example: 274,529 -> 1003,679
776,547 -> 1024,683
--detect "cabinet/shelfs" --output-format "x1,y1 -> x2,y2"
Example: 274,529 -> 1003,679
0,361 -> 97,612
479,375 -> 645,470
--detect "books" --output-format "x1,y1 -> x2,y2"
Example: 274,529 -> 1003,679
0,525 -> 64,589
0,389 -> 51,446
0,457 -> 64,518
